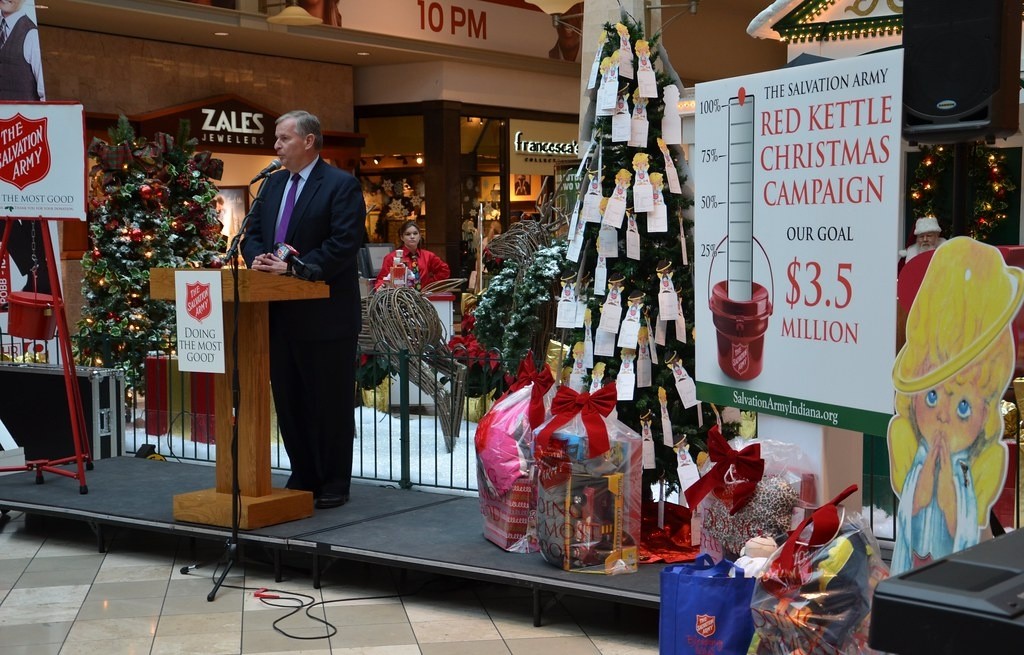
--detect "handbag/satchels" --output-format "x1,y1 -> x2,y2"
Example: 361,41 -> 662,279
658,552 -> 757,655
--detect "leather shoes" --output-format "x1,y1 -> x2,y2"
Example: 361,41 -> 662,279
317,492 -> 350,508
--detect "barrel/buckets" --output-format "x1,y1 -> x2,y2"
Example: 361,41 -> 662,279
708,282 -> 771,379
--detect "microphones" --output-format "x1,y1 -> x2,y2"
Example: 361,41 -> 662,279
250,159 -> 282,184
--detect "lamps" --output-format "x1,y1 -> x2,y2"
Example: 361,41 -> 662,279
257,0 -> 322,26
524,0 -> 584,36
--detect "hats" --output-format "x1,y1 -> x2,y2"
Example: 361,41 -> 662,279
914,214 -> 941,235
891,236 -> 1024,390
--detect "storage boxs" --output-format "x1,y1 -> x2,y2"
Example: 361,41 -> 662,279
481,432 -> 643,571
0,361 -> 127,461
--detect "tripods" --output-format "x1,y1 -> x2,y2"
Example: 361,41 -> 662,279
180,173 -> 276,601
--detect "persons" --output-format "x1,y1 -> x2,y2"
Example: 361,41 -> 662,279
898,215 -> 946,267
0,0 -> 53,311
239,111 -> 367,509
374,222 -> 451,291
475,221 -> 502,275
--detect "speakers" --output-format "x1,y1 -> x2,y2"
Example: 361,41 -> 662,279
900,0 -> 1022,146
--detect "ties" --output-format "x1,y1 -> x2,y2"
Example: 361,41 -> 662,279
0,18 -> 7,48
409,253 -> 422,291
273,173 -> 300,252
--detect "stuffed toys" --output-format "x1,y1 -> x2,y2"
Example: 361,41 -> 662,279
732,535 -> 779,579
